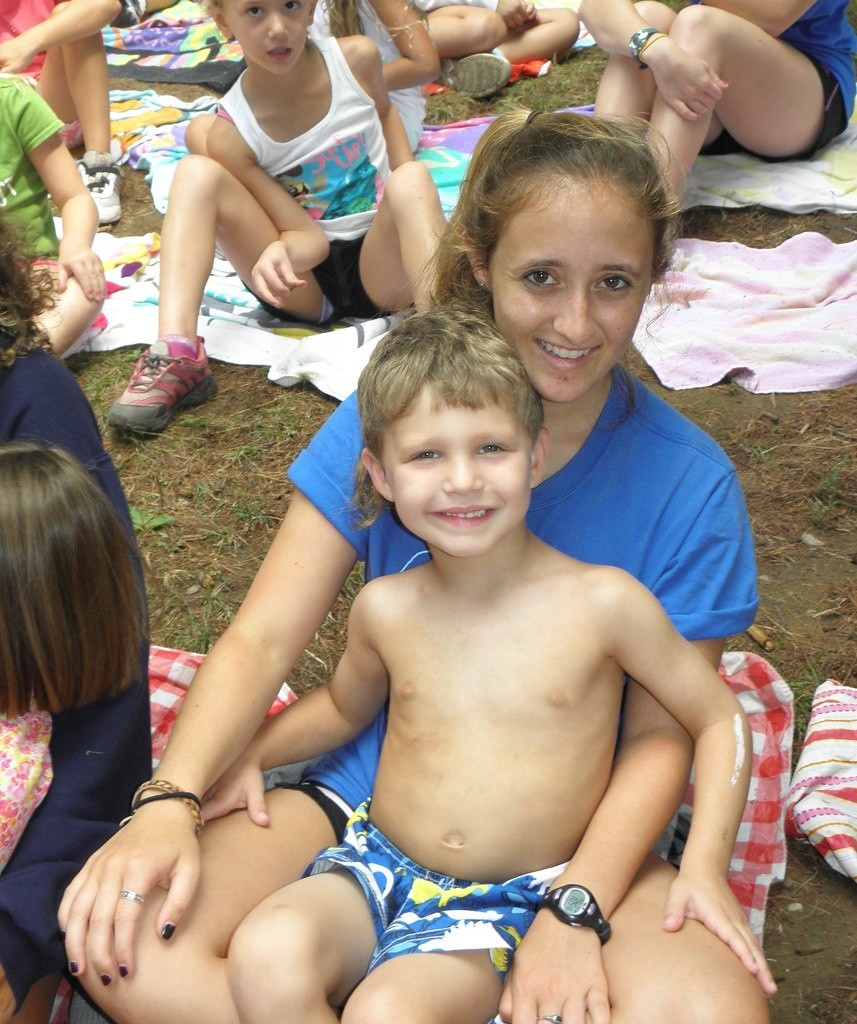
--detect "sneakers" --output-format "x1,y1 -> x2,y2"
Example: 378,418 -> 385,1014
75,159 -> 126,225
103,334 -> 218,438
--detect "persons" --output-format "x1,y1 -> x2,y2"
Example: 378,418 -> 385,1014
0,209 -> 141,1024
54,100 -> 764,1024
104,0 -> 456,436
0,1 -> 128,227
108,1 -> 179,29
400,0 -> 581,99
2,70 -> 110,368
574,0 -> 857,194
0,441 -> 148,875
194,298 -> 779,1024
304,0 -> 441,159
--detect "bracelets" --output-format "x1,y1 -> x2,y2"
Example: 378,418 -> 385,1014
120,779 -> 204,836
638,34 -> 667,64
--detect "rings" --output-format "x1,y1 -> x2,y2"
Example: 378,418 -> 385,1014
120,889 -> 145,904
536,1014 -> 563,1024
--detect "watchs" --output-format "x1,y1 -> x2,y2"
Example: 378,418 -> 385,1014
629,28 -> 659,69
536,883 -> 612,947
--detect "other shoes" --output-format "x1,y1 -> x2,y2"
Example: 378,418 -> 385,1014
435,53 -> 512,100
